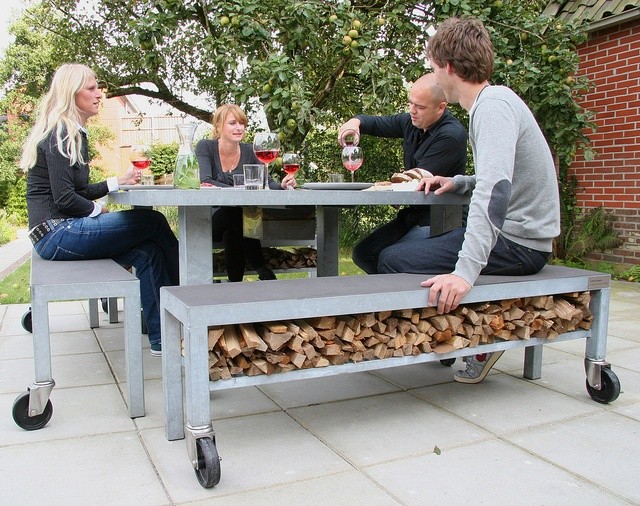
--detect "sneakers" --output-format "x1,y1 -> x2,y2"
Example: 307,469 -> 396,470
151,342 -> 162,357
454,351 -> 504,384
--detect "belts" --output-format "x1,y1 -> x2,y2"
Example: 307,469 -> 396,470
28,218 -> 65,246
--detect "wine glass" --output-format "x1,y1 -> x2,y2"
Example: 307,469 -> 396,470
281,153 -> 302,190
341,146 -> 364,183
253,133 -> 280,189
130,142 -> 151,186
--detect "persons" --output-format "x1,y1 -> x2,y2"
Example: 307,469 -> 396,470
337,73 -> 466,274
196,104 -> 297,281
20,64 -> 178,357
378,16 -> 560,383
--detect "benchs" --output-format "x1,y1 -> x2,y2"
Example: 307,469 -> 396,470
12,247 -> 146,431
160,264 -> 621,489
102,240 -> 317,323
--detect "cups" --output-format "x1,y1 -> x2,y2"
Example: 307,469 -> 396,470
233,173 -> 244,188
243,163 -> 264,190
328,173 -> 344,183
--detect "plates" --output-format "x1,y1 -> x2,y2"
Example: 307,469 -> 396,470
302,182 -> 372,191
118,184 -> 176,190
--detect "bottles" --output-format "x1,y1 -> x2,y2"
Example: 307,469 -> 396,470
174,123 -> 201,189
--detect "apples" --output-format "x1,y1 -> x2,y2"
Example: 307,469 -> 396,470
542,49 -> 548,56
348,29 -> 358,40
343,46 -> 351,56
541,45 -> 548,50
548,55 -> 557,64
542,54 -> 550,61
353,20 -> 361,31
278,132 -> 287,140
520,33 -> 527,41
378,19 -> 385,26
328,15 -> 338,23
269,79 -> 277,86
351,41 -> 358,49
221,16 -> 230,26
566,76 -> 576,86
492,1 -> 503,11
263,84 -> 271,93
276,157 -> 283,167
232,17 -> 240,26
287,119 -> 295,130
343,36 -> 352,45
292,101 -> 302,110
506,59 -> 514,66
